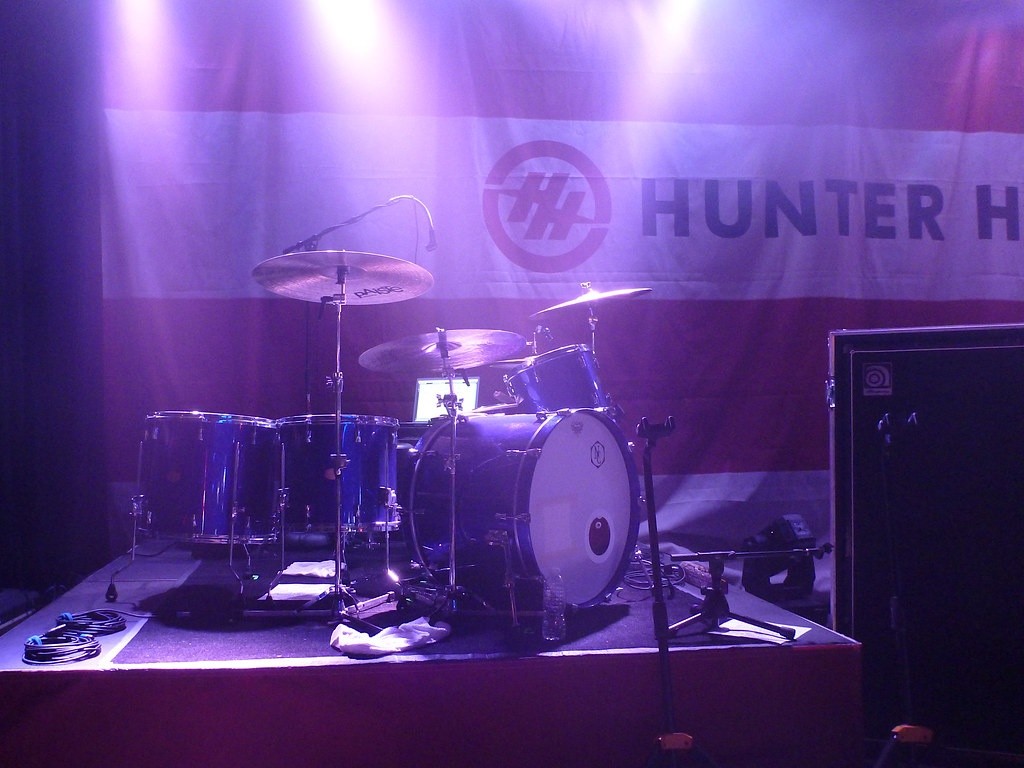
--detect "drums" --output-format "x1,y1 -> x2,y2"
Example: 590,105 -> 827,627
410,410 -> 639,621
505,344 -> 621,425
274,413 -> 399,535
131,410 -> 284,544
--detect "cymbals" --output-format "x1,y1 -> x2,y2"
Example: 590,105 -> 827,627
491,357 -> 534,370
359,329 -> 526,377
251,250 -> 435,304
529,285 -> 650,318
